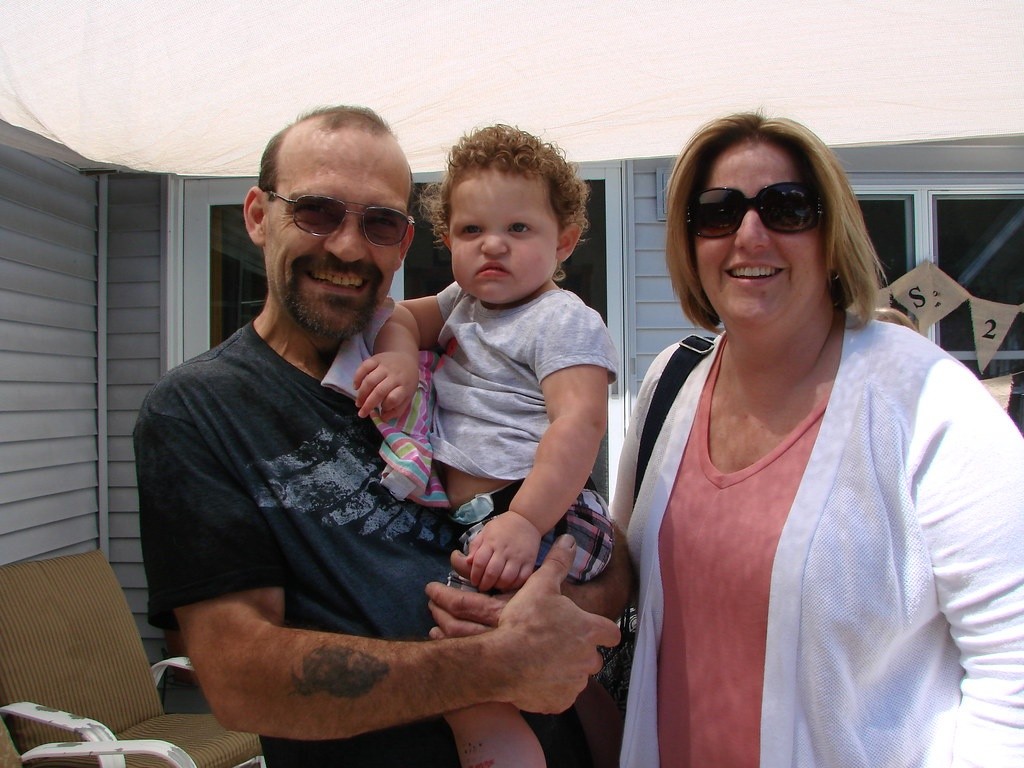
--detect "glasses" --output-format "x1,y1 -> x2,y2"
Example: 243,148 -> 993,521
267,191 -> 416,247
684,182 -> 825,238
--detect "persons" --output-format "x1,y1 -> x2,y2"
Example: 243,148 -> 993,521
572,106 -> 1024,768
133,105 -> 633,768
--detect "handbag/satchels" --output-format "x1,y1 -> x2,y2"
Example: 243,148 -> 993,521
591,605 -> 640,716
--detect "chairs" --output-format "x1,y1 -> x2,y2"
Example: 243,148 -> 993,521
0,548 -> 266,768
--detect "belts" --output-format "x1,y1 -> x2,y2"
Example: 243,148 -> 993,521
457,474 -> 598,537
